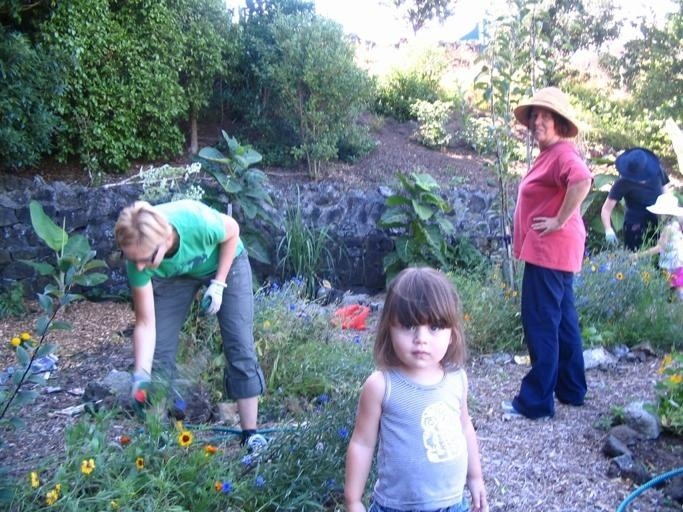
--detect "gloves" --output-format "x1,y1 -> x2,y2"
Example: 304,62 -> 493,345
200,278 -> 228,315
605,228 -> 620,249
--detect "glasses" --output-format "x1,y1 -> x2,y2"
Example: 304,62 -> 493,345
118,244 -> 159,264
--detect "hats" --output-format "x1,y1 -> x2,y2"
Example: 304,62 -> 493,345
513,86 -> 579,138
614,147 -> 661,183
645,192 -> 683,217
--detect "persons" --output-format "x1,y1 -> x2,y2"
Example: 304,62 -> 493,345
627,193 -> 682,298
113,197 -> 267,452
343,266 -> 489,512
505,85 -> 586,424
598,147 -> 668,251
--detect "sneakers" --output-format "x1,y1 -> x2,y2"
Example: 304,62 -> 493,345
241,434 -> 269,457
